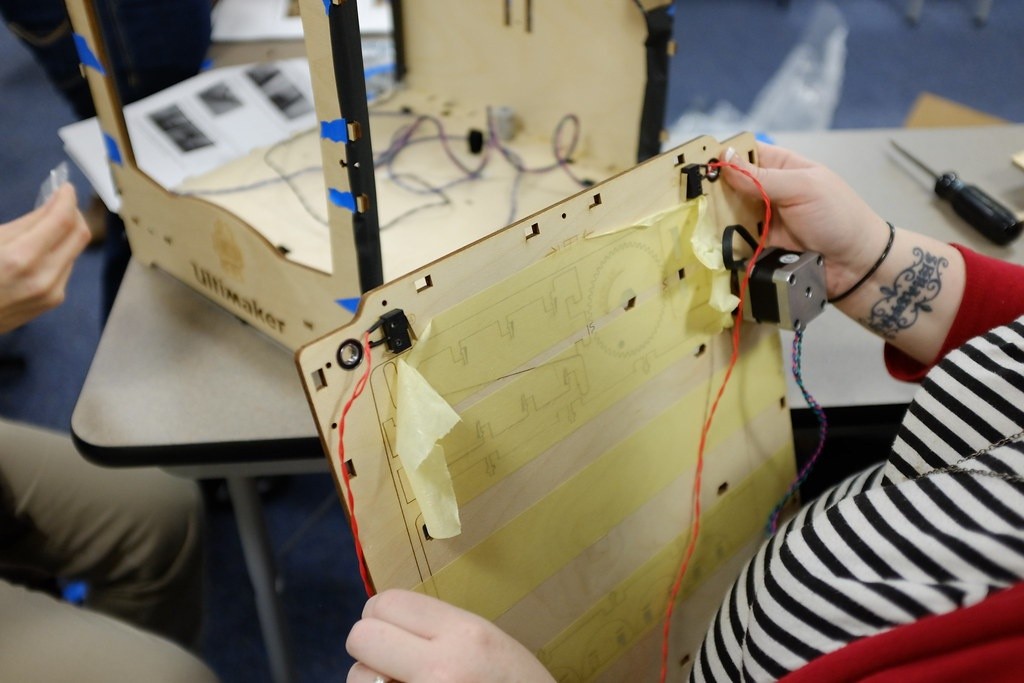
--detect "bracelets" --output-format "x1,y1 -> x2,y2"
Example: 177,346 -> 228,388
827,222 -> 893,303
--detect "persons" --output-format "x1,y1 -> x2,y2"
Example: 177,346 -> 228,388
0,0 -> 219,334
344,142 -> 1024,683
0,182 -> 220,683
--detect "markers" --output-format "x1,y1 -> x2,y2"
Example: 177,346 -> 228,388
974,0 -> 994,27
908,0 -> 925,26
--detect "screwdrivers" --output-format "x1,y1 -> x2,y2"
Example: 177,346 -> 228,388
889,140 -> 1024,245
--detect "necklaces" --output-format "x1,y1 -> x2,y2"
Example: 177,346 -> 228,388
915,430 -> 1024,481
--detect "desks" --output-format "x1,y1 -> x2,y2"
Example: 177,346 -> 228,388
67,30 -> 1022,682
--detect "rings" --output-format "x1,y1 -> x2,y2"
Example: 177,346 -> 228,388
374,676 -> 391,683
